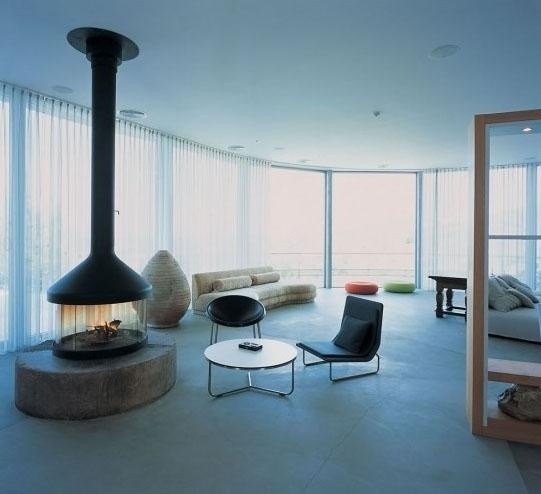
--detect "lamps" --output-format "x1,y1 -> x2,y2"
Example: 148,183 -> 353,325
120,107 -> 147,120
54,86 -> 76,95
230,145 -> 247,152
429,41 -> 461,63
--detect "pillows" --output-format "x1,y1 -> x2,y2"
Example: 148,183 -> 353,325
208,273 -> 252,290
489,271 -> 540,311
249,269 -> 282,284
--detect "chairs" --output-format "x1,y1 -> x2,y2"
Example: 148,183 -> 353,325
294,295 -> 384,380
206,294 -> 266,347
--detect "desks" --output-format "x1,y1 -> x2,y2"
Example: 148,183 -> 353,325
430,273 -> 469,323
203,338 -> 299,397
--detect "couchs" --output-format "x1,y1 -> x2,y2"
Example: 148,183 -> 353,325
188,264 -> 317,317
487,304 -> 541,345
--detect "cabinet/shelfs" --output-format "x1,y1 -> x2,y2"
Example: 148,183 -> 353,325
466,109 -> 541,446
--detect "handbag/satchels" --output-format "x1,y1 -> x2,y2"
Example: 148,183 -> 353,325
498,384 -> 540,422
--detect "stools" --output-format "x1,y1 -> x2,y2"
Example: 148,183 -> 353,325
384,279 -> 417,294
344,281 -> 381,296
